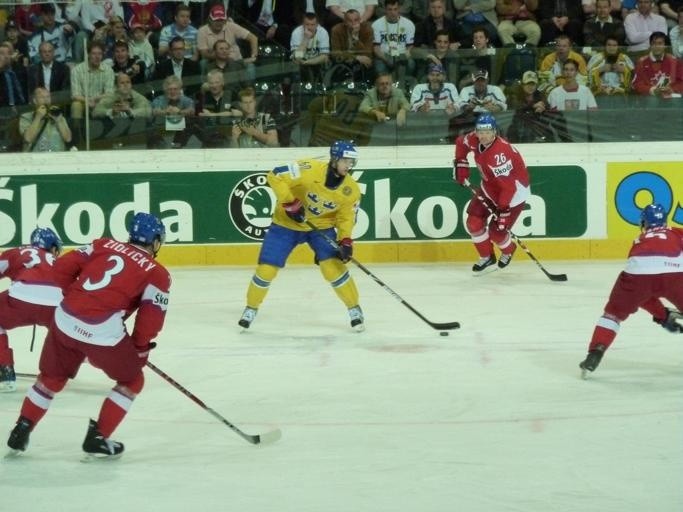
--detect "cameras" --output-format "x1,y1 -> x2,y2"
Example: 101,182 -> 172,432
232,118 -> 253,129
44,103 -> 62,117
475,99 -> 484,105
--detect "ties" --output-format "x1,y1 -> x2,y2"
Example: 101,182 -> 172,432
260,0 -> 271,25
7,70 -> 24,105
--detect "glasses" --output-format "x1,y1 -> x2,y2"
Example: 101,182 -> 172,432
173,47 -> 184,51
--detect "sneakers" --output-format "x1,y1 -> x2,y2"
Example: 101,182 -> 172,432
472,254 -> 497,272
0,366 -> 16,381
579,343 -> 608,371
7,415 -> 33,452
238,306 -> 257,328
82,420 -> 124,456
348,304 -> 363,327
498,247 -> 516,268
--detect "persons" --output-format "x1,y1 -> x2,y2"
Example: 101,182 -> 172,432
375,0 -> 412,18
149,75 -> 196,148
538,34 -> 588,98
414,0 -> 464,49
582,0 -> 621,19
326,0 -> 378,23
453,0 -> 498,41
587,35 -> 635,110
197,8 -> 258,72
410,30 -> 463,66
631,32 -> 683,111
238,139 -> 365,333
535,0 -> 586,48
157,5 -> 198,62
507,71 -> 548,114
289,13 -> 330,65
28,42 -> 71,90
71,41 -> 114,145
458,29 -> 504,84
459,68 -> 507,114
129,23 -> 154,79
19,88 -> 72,152
291,0 -> 330,32
583,1 -> 626,50
330,9 -> 373,68
193,69 -> 244,117
358,72 -> 410,128
299,88 -> 373,146
129,0 -> 162,32
413,0 -> 456,22
497,1 -> 541,47
0,45 -> 29,107
4,212 -> 172,462
456,113 -> 528,277
580,205 -> 683,381
0,227 -> 64,394
0,0 -> 129,61
246,0 -> 283,39
621,0 -> 658,19
670,8 -> 683,58
658,1 -> 683,30
91,72 -> 154,140
112,41 -> 146,84
371,0 -> 418,79
624,0 -> 668,63
546,59 -> 598,111
155,38 -> 202,80
410,66 -> 460,116
200,41 -> 255,100
230,89 -> 279,148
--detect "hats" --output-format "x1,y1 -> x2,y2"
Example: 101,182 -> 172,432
472,70 -> 489,81
132,23 -> 145,31
523,70 -> 537,84
109,16 -> 122,23
208,5 -> 227,21
114,39 -> 127,47
42,2 -> 55,10
8,21 -> 16,28
429,66 -> 445,74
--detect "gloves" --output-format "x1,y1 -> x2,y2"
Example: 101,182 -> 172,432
282,199 -> 305,223
335,238 -> 353,262
495,210 -> 511,231
652,308 -> 680,331
452,160 -> 471,186
132,340 -> 157,366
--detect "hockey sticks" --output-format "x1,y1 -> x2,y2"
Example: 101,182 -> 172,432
464,178 -> 567,282
300,215 -> 460,330
146,361 -> 284,445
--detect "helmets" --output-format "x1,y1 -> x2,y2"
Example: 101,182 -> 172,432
476,114 -> 497,133
130,213 -> 165,243
331,140 -> 357,158
30,226 -> 62,252
642,203 -> 667,228
